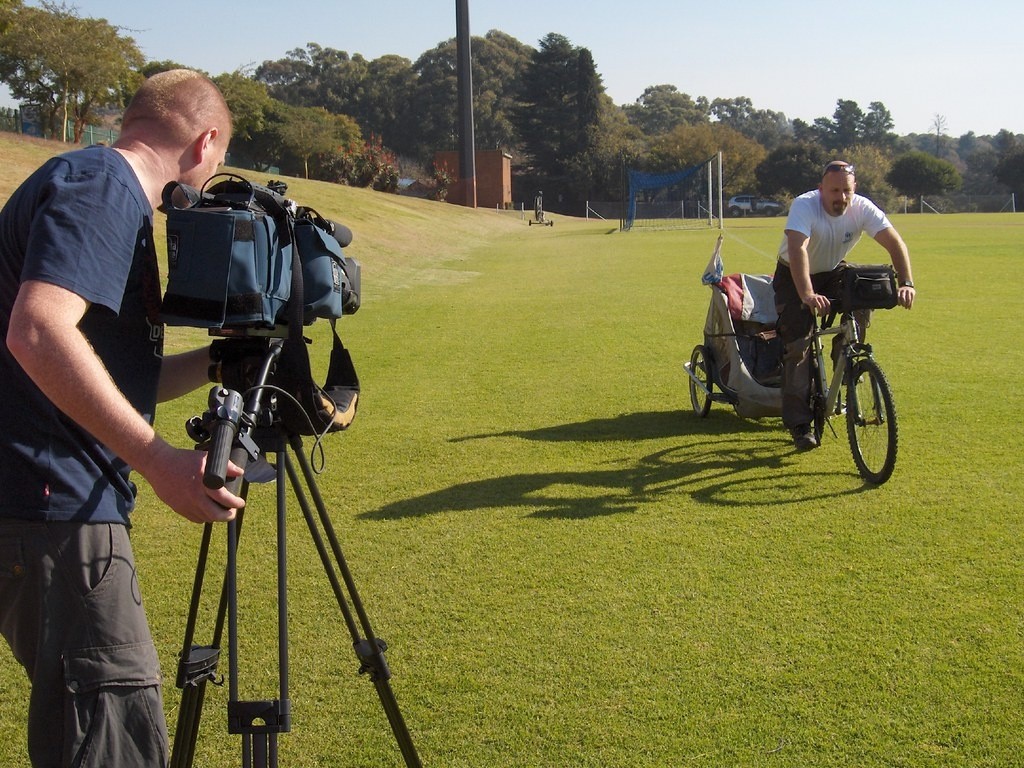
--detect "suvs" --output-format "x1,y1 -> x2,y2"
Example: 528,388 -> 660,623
728,194 -> 785,218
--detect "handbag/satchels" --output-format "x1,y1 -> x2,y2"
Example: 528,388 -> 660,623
828,262 -> 898,314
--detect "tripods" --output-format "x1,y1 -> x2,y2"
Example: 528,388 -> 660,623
176,430 -> 426,768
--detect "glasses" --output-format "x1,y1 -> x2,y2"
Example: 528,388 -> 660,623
822,165 -> 855,178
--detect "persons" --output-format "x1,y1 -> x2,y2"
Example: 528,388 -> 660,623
0,68 -> 251,768
773,160 -> 916,454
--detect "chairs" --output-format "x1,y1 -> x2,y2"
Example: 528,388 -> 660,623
722,270 -> 784,374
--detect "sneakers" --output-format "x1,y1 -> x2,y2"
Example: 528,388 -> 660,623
842,358 -> 864,385
789,423 -> 817,452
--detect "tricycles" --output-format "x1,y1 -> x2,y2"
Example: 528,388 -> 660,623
690,238 -> 912,487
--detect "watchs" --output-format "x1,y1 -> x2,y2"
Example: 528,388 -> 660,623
899,279 -> 914,287
